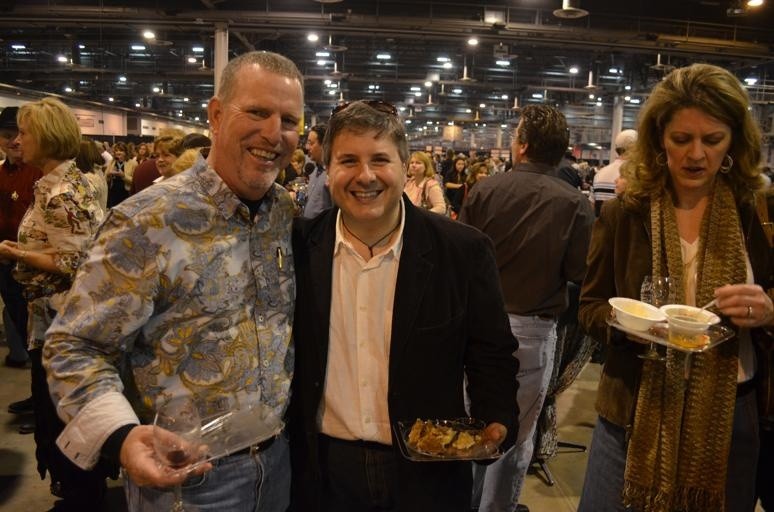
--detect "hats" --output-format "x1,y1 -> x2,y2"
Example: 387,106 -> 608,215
1,108 -> 19,129
616,129 -> 638,149
168,134 -> 211,154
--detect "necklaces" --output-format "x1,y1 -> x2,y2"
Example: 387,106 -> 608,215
339,215 -> 400,259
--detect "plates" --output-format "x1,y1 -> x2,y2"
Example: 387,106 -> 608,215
405,419 -> 482,458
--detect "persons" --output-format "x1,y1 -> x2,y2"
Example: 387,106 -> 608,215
577,63 -> 773,512
0,270 -> 29,369
558,130 -> 647,216
43,50 -> 306,510
738,147 -> 773,262
0,107 -> 43,435
452,103 -> 594,510
1,99 -> 105,511
281,123 -> 516,222
78,119 -> 210,208
291,98 -> 520,510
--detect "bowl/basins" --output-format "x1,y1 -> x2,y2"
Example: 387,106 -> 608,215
608,296 -> 665,332
659,303 -> 720,331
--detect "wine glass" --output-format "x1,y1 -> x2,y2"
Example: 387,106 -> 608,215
295,184 -> 312,216
152,393 -> 201,512
638,275 -> 677,361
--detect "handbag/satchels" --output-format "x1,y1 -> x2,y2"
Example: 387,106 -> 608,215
422,196 -> 449,219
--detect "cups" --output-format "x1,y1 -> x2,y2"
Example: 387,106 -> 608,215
452,416 -> 486,458
665,307 -> 712,349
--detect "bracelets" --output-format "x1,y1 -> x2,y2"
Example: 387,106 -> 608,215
760,221 -> 773,226
18,249 -> 27,264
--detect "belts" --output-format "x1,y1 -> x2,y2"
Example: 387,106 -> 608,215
228,434 -> 275,454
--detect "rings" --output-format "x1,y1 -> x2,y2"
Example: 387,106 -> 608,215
748,308 -> 754,320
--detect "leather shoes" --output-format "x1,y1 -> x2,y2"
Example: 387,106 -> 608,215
8,396 -> 33,414
20,423 -> 35,434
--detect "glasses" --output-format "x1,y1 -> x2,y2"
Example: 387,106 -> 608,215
333,100 -> 398,116
